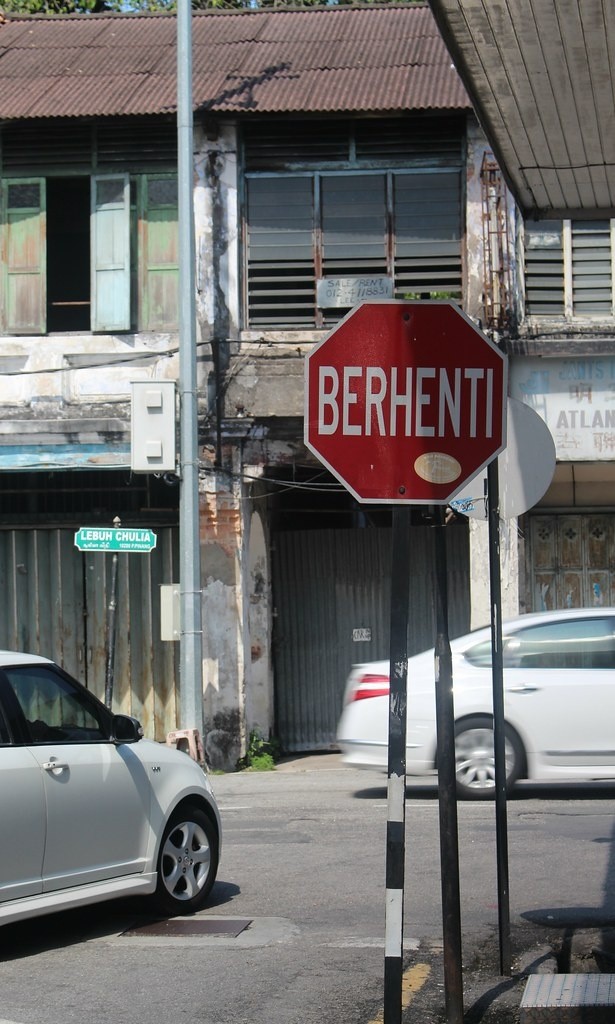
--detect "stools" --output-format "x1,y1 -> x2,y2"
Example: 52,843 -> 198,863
518,970 -> 615,1024
165,727 -> 206,762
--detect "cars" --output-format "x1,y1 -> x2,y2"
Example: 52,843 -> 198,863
0,647 -> 222,927
335,605 -> 615,804
